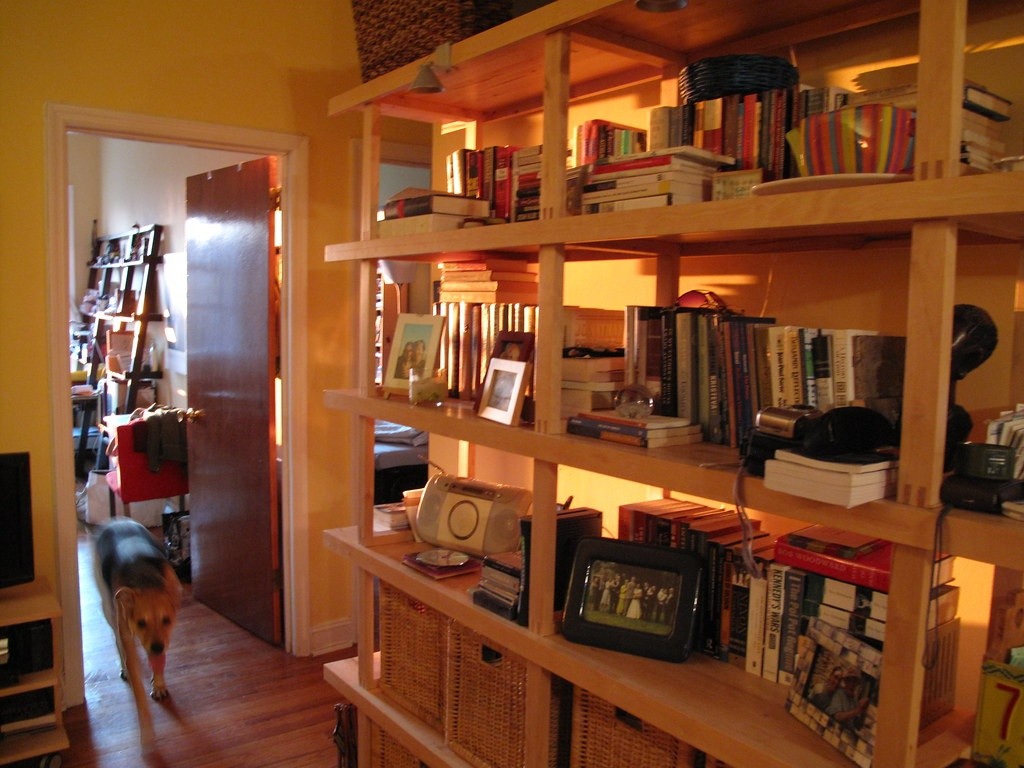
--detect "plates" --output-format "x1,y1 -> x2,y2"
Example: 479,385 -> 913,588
752,174 -> 914,193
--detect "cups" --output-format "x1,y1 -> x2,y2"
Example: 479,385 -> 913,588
408,369 -> 448,404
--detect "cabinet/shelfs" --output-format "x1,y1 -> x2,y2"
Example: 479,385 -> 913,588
323,0 -> 1024,768
0,575 -> 70,768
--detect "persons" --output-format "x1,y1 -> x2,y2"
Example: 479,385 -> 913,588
589,567 -> 676,624
394,340 -> 427,381
810,666 -> 874,731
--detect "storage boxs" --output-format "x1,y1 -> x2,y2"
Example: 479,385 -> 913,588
378,577 -> 448,738
570,684 -> 696,768
352,0 -> 514,84
445,618 -> 526,768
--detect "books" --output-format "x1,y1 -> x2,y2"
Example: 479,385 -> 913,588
472,450 -> 961,768
430,252 -> 907,447
377,77 -> 1015,239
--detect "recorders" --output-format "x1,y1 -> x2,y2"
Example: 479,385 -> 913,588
416,453 -> 533,559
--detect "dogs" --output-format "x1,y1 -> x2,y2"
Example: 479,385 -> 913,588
93,517 -> 183,760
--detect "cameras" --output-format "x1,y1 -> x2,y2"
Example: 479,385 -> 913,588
755,404 -> 825,440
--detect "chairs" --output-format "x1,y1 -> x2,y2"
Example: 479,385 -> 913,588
105,424 -> 189,518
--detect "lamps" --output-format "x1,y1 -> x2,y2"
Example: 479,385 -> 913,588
407,43 -> 457,94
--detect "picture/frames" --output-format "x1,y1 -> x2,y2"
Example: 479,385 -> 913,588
562,536 -> 705,664
785,616 -> 882,768
474,331 -> 535,414
383,313 -> 446,400
477,358 -> 533,426
